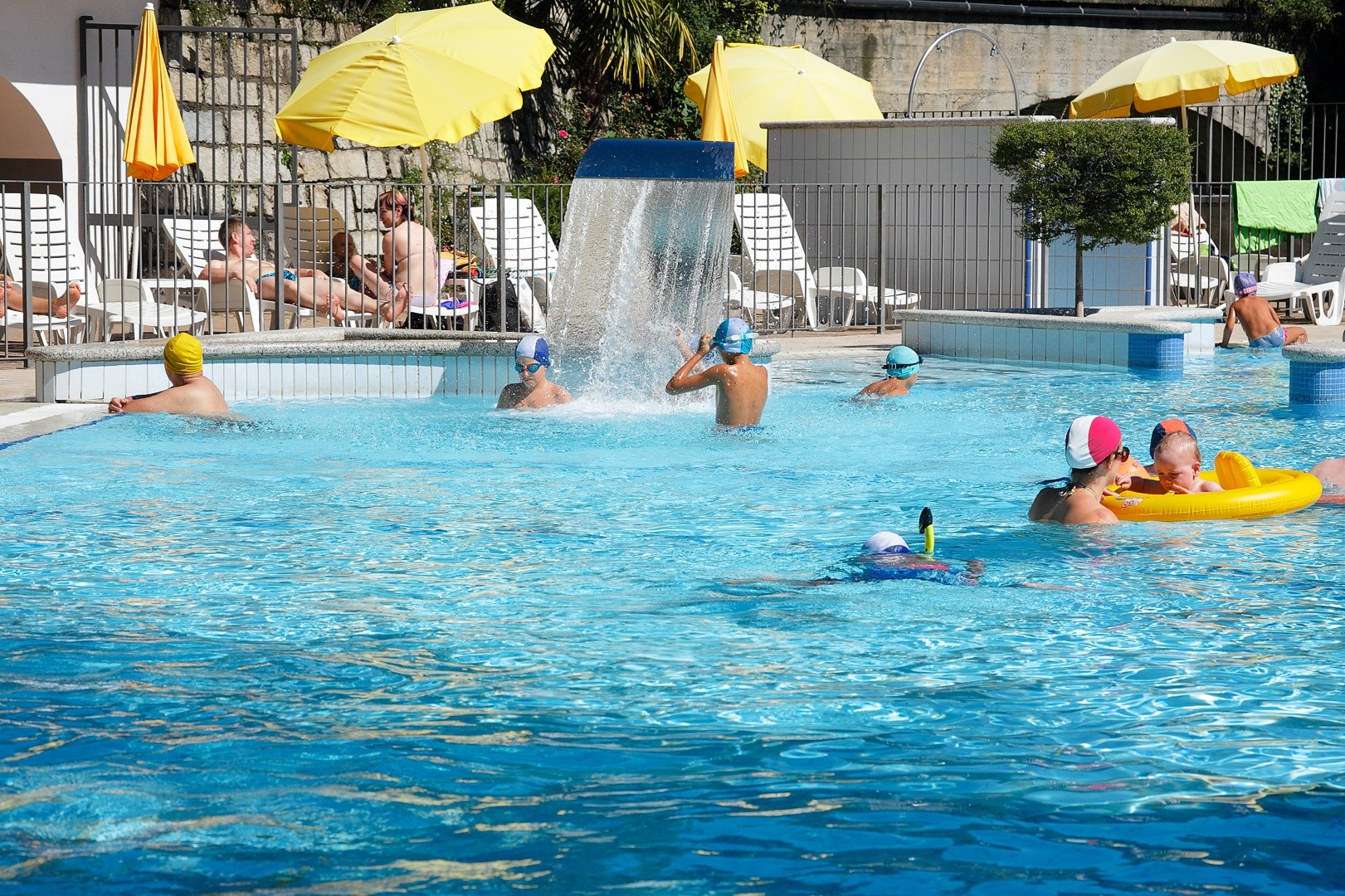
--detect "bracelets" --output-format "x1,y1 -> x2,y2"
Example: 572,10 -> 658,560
305,268 -> 312,277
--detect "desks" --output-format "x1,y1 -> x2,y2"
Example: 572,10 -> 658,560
444,278 -> 506,331
133,278 -> 212,333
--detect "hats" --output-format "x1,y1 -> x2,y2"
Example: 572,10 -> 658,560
887,346 -> 919,378
515,335 -> 550,367
1065,415 -> 1122,468
1234,273 -> 1257,296
715,317 -> 752,354
163,333 -> 204,375
861,532 -> 911,553
1150,419 -> 1197,459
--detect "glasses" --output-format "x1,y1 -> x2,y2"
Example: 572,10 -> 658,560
882,355 -> 924,369
1116,446 -> 1130,463
514,363 -> 541,373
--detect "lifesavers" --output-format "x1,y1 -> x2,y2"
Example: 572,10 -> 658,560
1098,452 -> 1323,520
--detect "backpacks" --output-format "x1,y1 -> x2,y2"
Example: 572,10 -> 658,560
478,276 -> 522,332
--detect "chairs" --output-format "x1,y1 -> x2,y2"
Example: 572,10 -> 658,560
725,255 -> 796,333
1167,194 -> 1345,325
0,193 -> 559,347
733,193 -> 922,328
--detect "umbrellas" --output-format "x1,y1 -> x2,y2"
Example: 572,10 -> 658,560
274,1 -> 557,234
683,44 -> 886,172
700,36 -> 750,179
123,2 -> 197,283
1068,37 -> 1300,264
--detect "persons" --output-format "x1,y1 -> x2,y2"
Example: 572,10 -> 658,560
1028,416 -> 1130,523
855,346 -> 924,397
197,218 -> 413,321
861,532 -> 912,554
1217,273 -> 1308,349
665,317 -> 768,427
325,189 -> 438,308
497,336 -> 573,408
0,274 -> 82,318
1115,419 -> 1225,495
108,332 -> 229,415
